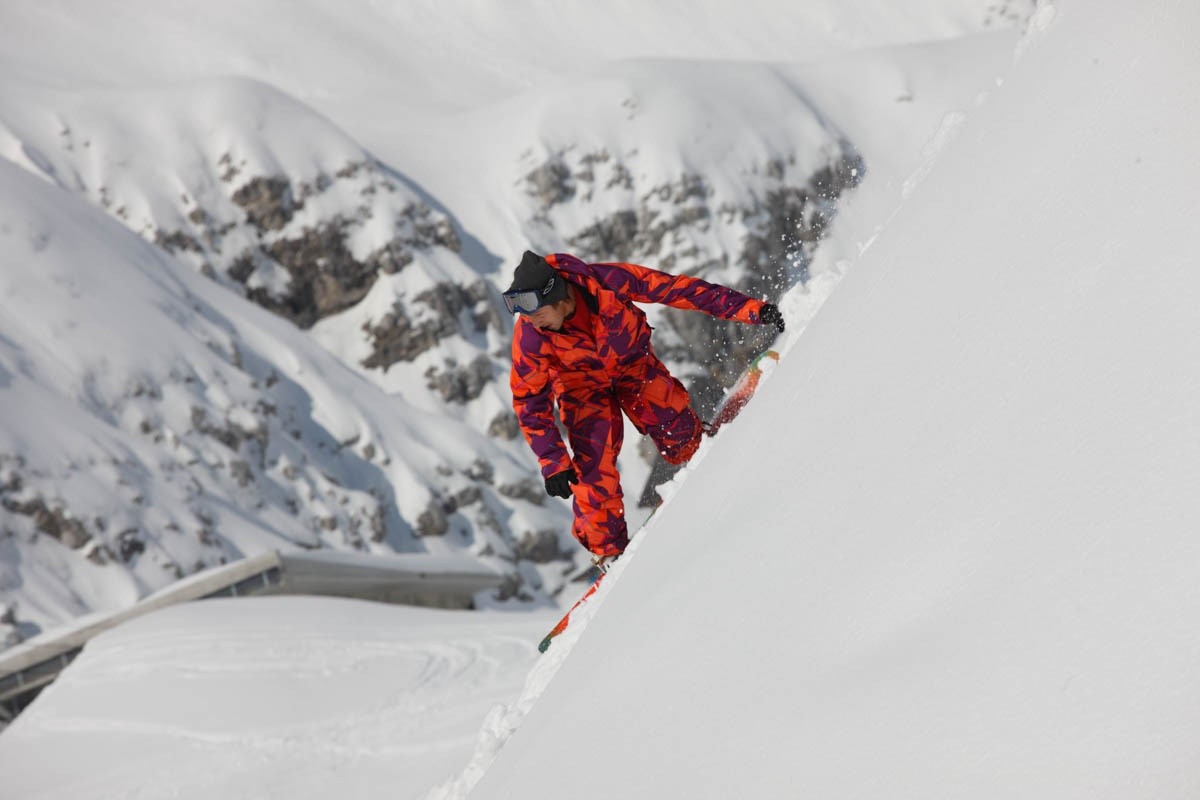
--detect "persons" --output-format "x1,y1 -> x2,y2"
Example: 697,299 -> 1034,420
501,249 -> 785,571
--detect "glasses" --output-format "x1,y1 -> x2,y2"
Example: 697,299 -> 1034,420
502,271 -> 557,318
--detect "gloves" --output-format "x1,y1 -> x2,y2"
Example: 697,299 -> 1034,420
544,467 -> 578,500
760,303 -> 786,335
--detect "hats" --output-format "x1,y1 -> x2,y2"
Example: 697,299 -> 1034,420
508,247 -> 567,310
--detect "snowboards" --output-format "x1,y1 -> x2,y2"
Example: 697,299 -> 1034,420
538,348 -> 780,654
